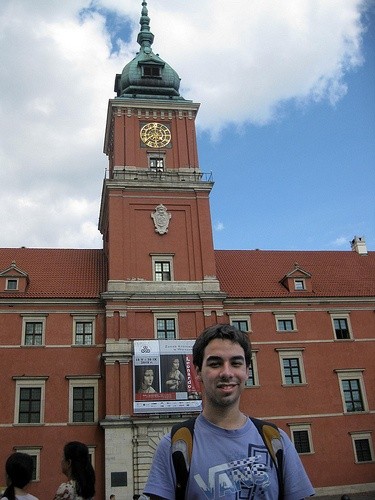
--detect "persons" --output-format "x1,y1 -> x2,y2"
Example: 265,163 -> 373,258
133,494 -> 140,500
142,323 -> 316,500
0,452 -> 39,500
110,495 -> 115,500
53,441 -> 96,500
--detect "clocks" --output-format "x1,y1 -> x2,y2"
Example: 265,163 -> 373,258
140,122 -> 172,148
